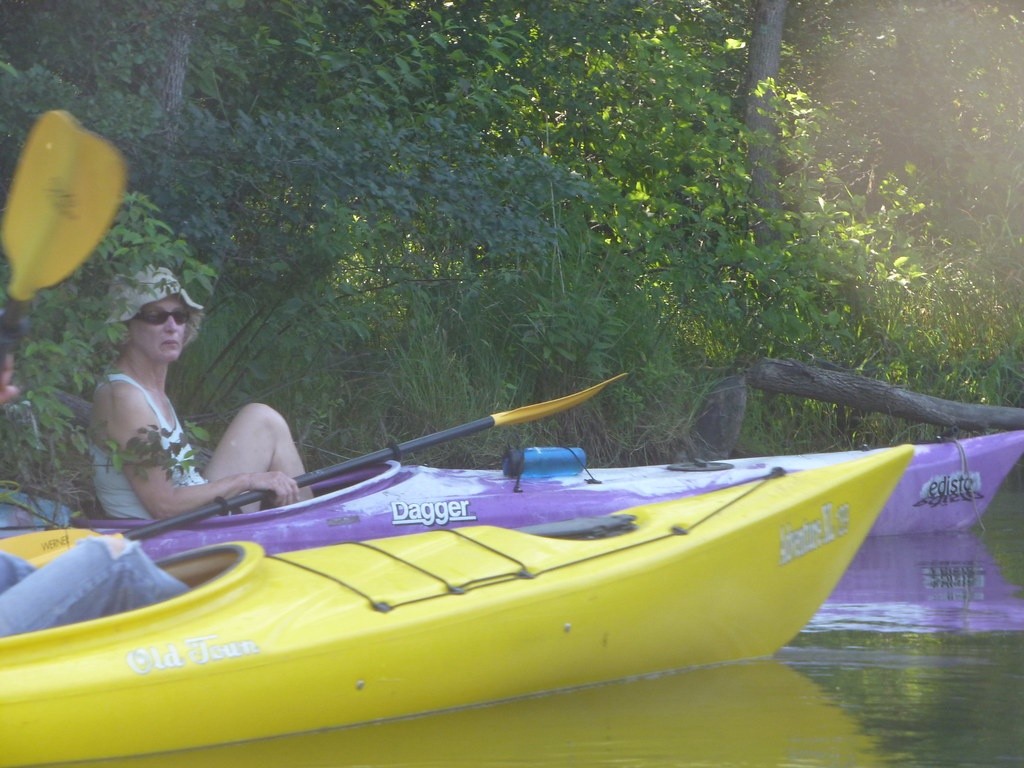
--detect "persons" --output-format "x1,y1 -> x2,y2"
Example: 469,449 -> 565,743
0,349 -> 194,637
87,265 -> 315,522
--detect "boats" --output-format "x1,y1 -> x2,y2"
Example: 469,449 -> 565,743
67,433 -> 1021,535
1,442 -> 915,768
804,532 -> 1024,633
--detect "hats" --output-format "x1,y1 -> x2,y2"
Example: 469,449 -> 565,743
102,263 -> 204,325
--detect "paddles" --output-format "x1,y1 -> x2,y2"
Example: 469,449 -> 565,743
1,109 -> 128,359
118,372 -> 634,540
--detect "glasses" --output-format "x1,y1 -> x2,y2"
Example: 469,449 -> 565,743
133,307 -> 189,325
